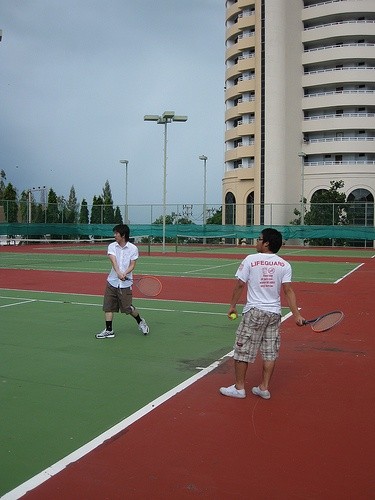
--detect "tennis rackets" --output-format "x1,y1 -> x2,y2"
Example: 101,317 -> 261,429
296,309 -> 345,334
120,275 -> 163,297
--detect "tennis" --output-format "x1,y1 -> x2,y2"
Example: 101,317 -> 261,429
230,312 -> 237,320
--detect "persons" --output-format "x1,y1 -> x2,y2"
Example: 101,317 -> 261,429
7,232 -> 15,245
89,233 -> 94,245
95,224 -> 149,339
219,228 -> 307,399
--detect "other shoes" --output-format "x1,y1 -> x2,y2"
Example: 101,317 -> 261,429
95,328 -> 115,339
252,385 -> 270,399
137,318 -> 149,335
219,384 -> 246,399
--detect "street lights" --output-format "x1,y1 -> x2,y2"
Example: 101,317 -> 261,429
298,152 -> 307,246
120,159 -> 129,224
199,154 -> 208,244
143,111 -> 188,252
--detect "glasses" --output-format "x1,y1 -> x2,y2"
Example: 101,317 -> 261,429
257,237 -> 263,242
